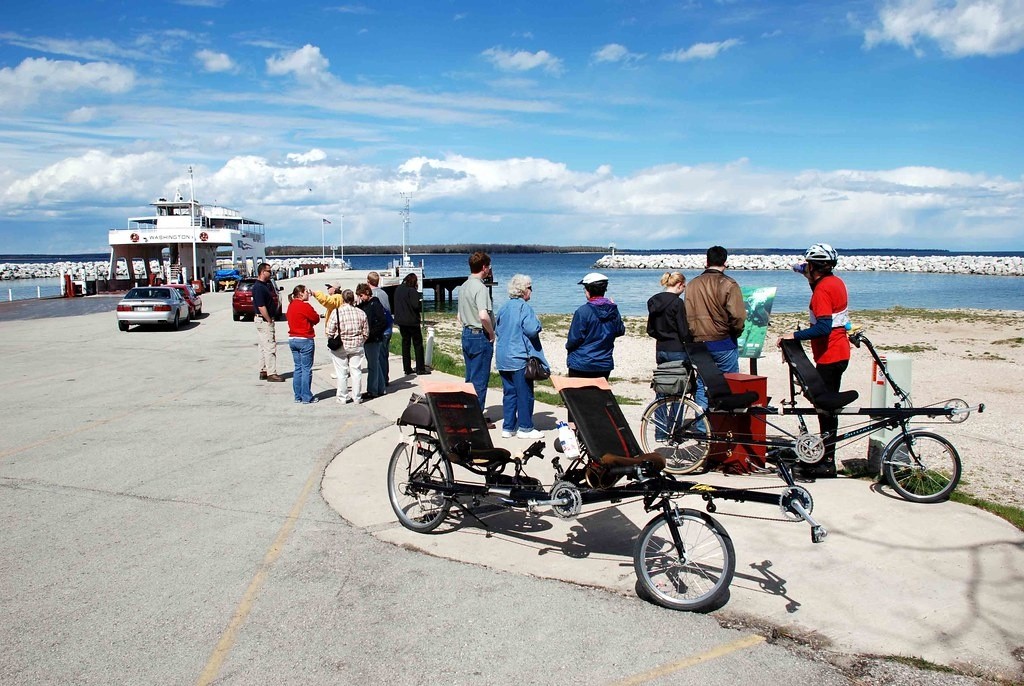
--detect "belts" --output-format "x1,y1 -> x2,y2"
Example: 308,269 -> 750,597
255,314 -> 263,317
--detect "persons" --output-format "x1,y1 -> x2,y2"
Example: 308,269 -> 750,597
307,282 -> 350,379
566,273 -> 625,422
287,285 -> 321,403
177,270 -> 184,284
353,271 -> 393,400
255,263 -> 285,381
457,251 -> 496,428
327,290 -> 369,405
396,273 -> 430,374
683,246 -> 746,441
496,274 -> 550,439
647,272 -> 695,442
189,276 -> 193,285
778,245 -> 851,480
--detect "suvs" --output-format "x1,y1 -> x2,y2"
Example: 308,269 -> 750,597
232,276 -> 284,321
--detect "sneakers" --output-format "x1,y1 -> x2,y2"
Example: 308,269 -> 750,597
502,430 -> 516,437
485,417 -> 496,429
295,396 -> 319,403
517,429 -> 545,439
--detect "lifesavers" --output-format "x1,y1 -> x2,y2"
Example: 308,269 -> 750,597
199,232 -> 208,241
130,233 -> 139,242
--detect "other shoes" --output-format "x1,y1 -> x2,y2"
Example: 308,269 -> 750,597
260,371 -> 285,382
804,463 -> 837,477
331,374 -> 348,378
405,370 -> 416,375
417,369 -> 431,374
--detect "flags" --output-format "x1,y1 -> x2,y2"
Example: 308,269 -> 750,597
323,219 -> 331,225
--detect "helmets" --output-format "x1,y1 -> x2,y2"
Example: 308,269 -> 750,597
806,243 -> 838,261
577,273 -> 609,289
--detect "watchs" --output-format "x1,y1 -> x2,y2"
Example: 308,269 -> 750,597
489,339 -> 493,343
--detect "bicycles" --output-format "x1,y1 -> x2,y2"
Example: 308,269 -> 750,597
389,374 -> 826,611
639,325 -> 985,505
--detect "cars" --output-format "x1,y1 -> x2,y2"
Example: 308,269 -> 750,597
116,287 -> 191,331
161,284 -> 202,319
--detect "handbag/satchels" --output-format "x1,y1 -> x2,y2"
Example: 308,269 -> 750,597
653,360 -> 691,394
525,357 -> 550,381
327,335 -> 343,351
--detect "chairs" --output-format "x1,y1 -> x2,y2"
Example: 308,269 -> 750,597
417,380 -> 512,464
682,341 -> 760,408
778,339 -> 859,410
550,375 -> 666,474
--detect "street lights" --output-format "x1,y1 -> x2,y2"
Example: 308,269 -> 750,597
188,165 -> 199,281
341,215 -> 344,261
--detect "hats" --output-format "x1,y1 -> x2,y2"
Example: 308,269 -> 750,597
325,280 -> 341,289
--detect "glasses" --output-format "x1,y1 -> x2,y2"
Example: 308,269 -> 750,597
264,270 -> 271,273
527,286 -> 531,289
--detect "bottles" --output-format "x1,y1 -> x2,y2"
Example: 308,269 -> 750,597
793,262 -> 809,275
557,421 -> 581,460
